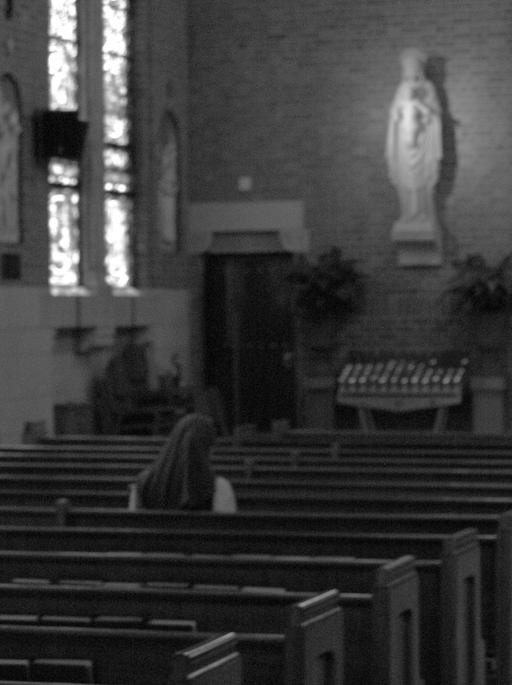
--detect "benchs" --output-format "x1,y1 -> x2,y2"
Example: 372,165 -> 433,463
0,550 -> 420,684
0,578 -> 346,685
0,524 -> 486,685
0,614 -> 243,684
0,437 -> 512,506
0,502 -> 512,685
0,659 -> 93,684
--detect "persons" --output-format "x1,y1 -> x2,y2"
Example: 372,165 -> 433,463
128,415 -> 237,514
385,47 -> 446,224
396,84 -> 432,149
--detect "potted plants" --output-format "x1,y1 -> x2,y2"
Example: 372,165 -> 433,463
290,247 -> 373,349
440,253 -> 512,348
